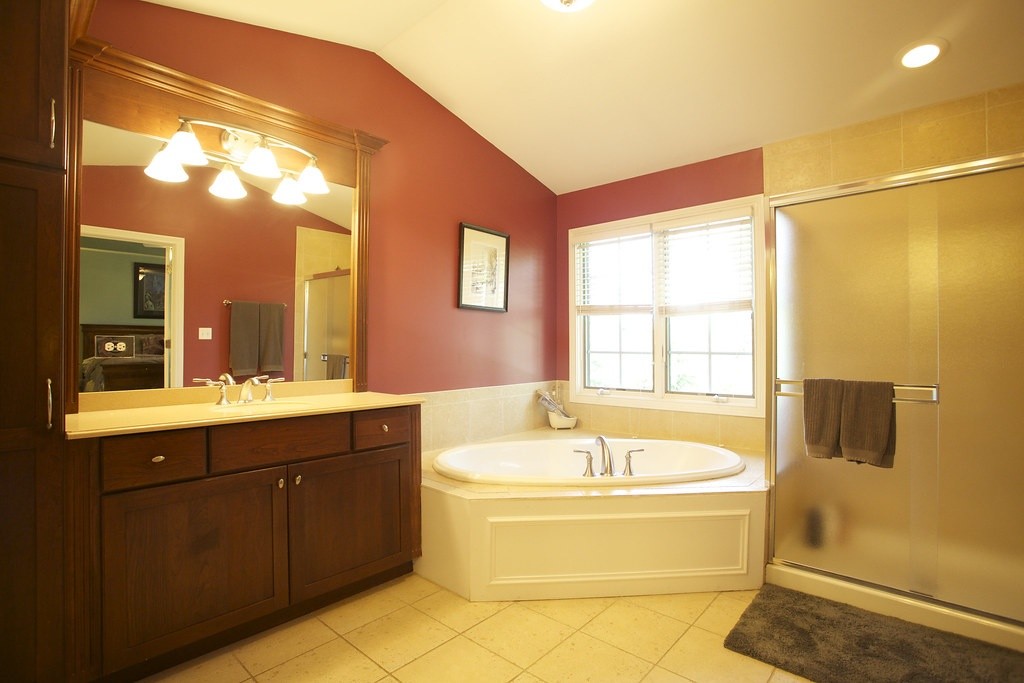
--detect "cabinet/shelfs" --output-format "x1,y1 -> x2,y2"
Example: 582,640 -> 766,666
0,1 -> 423,682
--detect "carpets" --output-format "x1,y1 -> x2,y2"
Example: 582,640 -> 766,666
724,584 -> 1024,683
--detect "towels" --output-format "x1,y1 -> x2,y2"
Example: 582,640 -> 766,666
840,381 -> 896,468
229,300 -> 284,375
803,376 -> 844,459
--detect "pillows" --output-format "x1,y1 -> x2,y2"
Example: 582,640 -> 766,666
141,335 -> 164,355
94,333 -> 135,358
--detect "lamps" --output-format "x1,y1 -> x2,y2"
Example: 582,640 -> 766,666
165,117 -> 330,195
144,142 -> 308,205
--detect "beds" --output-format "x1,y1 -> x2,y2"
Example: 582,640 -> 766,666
81,324 -> 164,392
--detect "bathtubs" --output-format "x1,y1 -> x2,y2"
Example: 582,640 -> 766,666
413,425 -> 767,602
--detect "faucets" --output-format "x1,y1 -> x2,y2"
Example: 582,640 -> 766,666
240,377 -> 260,400
219,372 -> 237,385
595,434 -> 616,477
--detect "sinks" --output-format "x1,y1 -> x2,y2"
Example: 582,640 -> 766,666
65,393 -> 422,444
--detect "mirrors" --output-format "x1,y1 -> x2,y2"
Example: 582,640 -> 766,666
78,65 -> 357,394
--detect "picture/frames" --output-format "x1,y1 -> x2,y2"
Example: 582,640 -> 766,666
134,262 -> 165,319
458,222 -> 510,312
